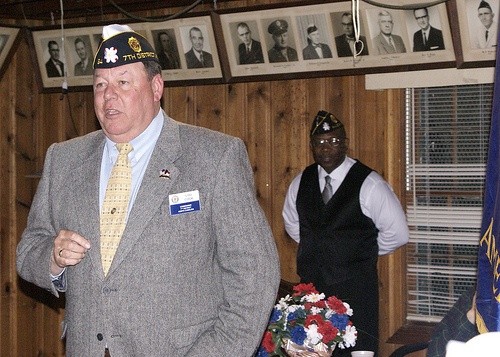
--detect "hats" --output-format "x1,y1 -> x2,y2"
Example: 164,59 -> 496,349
311,110 -> 344,135
479,0 -> 489,8
268,19 -> 288,34
92,24 -> 160,69
307,25 -> 317,34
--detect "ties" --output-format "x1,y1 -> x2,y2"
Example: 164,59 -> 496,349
246,45 -> 249,55
388,38 -> 394,49
168,53 -> 176,69
100,143 -> 134,277
423,32 -> 427,45
485,31 -> 488,41
322,176 -> 333,205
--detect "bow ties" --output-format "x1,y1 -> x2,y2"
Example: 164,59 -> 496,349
313,45 -> 321,49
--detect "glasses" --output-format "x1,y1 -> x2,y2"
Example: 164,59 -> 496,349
416,15 -> 428,19
313,138 -> 344,147
50,49 -> 59,51
341,22 -> 352,26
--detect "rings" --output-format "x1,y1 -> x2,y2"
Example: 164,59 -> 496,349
58,248 -> 63,257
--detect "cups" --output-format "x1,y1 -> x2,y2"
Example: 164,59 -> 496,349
351,351 -> 374,357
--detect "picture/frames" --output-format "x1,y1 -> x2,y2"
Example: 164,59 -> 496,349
0,0 -> 500,93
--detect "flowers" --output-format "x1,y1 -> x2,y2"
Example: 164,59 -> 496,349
260,284 -> 358,357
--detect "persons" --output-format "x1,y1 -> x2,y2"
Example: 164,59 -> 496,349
237,6 -> 446,65
44,27 -> 214,78
16,24 -> 281,356
473,0 -> 496,47
282,110 -> 409,357
